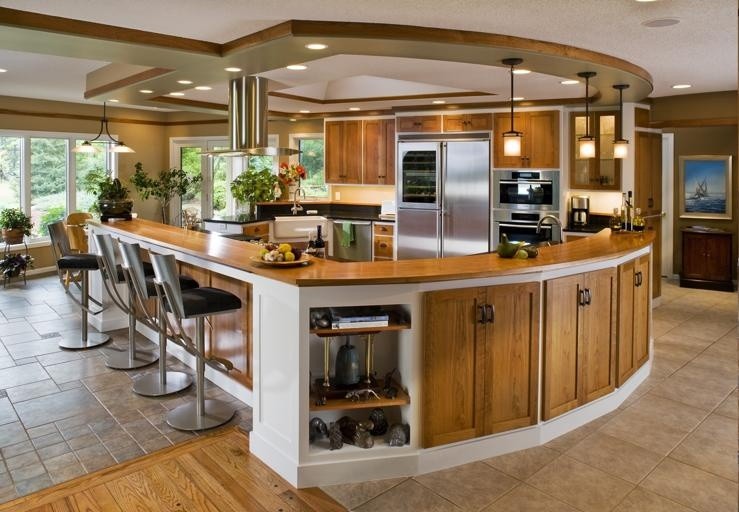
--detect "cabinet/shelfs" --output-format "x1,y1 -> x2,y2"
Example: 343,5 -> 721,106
309,321 -> 411,411
325,120 -> 395,185
396,113 -> 494,131
634,131 -> 662,300
494,110 -> 560,169
242,219 -> 271,244
679,225 -> 735,292
371,220 -> 397,263
4,241 -> 28,288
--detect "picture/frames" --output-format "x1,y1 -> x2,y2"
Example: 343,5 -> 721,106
678,153 -> 733,221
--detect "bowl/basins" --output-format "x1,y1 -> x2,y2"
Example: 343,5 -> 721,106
316,376 -> 379,399
495,244 -> 519,257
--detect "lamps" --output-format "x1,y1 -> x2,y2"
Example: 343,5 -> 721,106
69,98 -> 137,155
612,84 -> 629,158
501,57 -> 524,157
576,72 -> 596,159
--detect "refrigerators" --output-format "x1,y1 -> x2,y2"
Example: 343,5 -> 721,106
393,132 -> 491,261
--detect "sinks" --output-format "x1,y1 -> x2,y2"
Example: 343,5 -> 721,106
274,215 -> 328,238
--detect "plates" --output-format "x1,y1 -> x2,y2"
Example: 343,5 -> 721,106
250,254 -> 315,265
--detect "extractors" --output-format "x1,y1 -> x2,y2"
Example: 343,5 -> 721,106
197,77 -> 303,159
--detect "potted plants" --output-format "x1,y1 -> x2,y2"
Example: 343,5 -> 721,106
95,177 -> 136,223
229,165 -> 285,217
0,208 -> 35,245
0,251 -> 37,280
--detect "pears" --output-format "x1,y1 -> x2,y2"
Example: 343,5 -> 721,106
279,244 -> 291,252
284,252 -> 295,261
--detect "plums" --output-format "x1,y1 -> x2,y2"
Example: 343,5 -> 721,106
292,247 -> 302,260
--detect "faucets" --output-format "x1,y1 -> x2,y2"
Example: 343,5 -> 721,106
291,188 -> 303,216
536,214 -> 564,242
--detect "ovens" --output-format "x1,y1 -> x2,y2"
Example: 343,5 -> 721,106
489,169 -> 561,253
331,219 -> 372,263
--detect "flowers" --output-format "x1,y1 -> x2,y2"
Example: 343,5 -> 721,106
277,163 -> 307,183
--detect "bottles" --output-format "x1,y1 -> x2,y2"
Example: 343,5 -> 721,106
608,191 -> 645,232
187,219 -> 205,231
314,225 -> 326,258
306,233 -> 318,256
334,346 -> 360,383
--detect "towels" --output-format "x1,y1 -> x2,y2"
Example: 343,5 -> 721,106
339,222 -> 355,249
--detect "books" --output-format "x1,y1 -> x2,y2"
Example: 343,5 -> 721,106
331,307 -> 389,329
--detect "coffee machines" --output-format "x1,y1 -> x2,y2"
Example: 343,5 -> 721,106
570,196 -> 591,229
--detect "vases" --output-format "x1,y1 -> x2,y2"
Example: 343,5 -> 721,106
275,178 -> 291,203
